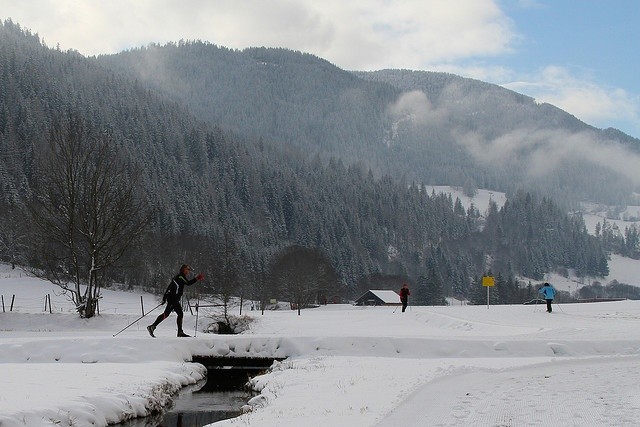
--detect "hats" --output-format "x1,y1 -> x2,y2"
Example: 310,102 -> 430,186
180,264 -> 194,271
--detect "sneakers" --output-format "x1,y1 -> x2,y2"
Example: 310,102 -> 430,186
147,325 -> 155,337
177,330 -> 190,337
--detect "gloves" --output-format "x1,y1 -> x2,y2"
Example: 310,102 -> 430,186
195,274 -> 204,280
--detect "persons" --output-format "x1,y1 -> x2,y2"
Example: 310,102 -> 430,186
399,284 -> 410,312
537,283 -> 556,313
147,264 -> 204,337
